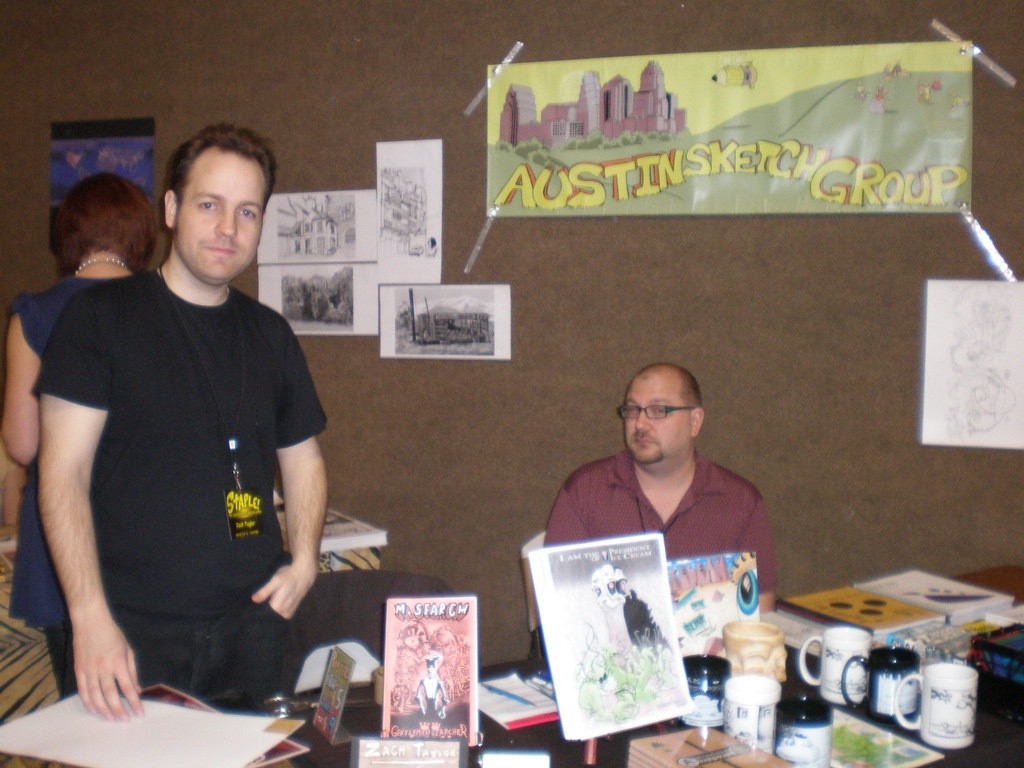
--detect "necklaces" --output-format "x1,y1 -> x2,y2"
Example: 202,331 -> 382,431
75,258 -> 126,276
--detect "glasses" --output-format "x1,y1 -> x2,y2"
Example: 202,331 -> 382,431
617,404 -> 694,419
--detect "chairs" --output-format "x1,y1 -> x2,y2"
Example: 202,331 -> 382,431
280,569 -> 455,705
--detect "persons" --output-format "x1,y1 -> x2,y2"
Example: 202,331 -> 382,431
29,120 -> 457,724
543,361 -> 778,615
1,169 -> 158,698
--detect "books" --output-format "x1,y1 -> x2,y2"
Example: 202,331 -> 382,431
783,568 -> 1024,685
629,707 -> 946,768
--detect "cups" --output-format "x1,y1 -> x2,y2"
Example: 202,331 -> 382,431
723,621 -> 787,682
893,663 -> 979,749
775,697 -> 834,768
682,654 -> 732,732
841,646 -> 920,719
799,626 -> 871,704
723,674 -> 781,754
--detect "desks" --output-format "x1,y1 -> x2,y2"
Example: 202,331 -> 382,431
246,565 -> 1024,768
320,506 -> 390,555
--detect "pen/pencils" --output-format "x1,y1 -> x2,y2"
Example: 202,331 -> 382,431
480,682 -> 535,707
